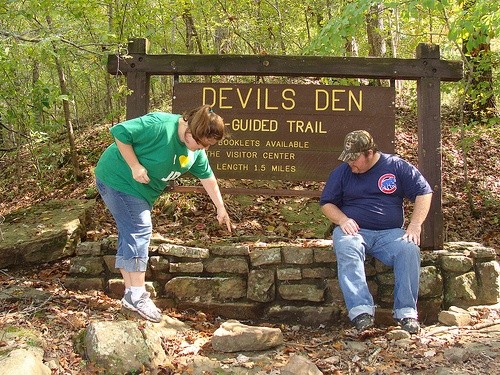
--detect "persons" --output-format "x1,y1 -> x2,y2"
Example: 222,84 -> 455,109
320,130 -> 434,334
93,104 -> 234,323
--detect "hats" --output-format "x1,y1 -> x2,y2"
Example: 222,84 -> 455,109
338,129 -> 374,163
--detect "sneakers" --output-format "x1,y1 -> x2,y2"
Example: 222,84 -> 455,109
354,314 -> 374,333
394,318 -> 420,333
121,291 -> 162,323
